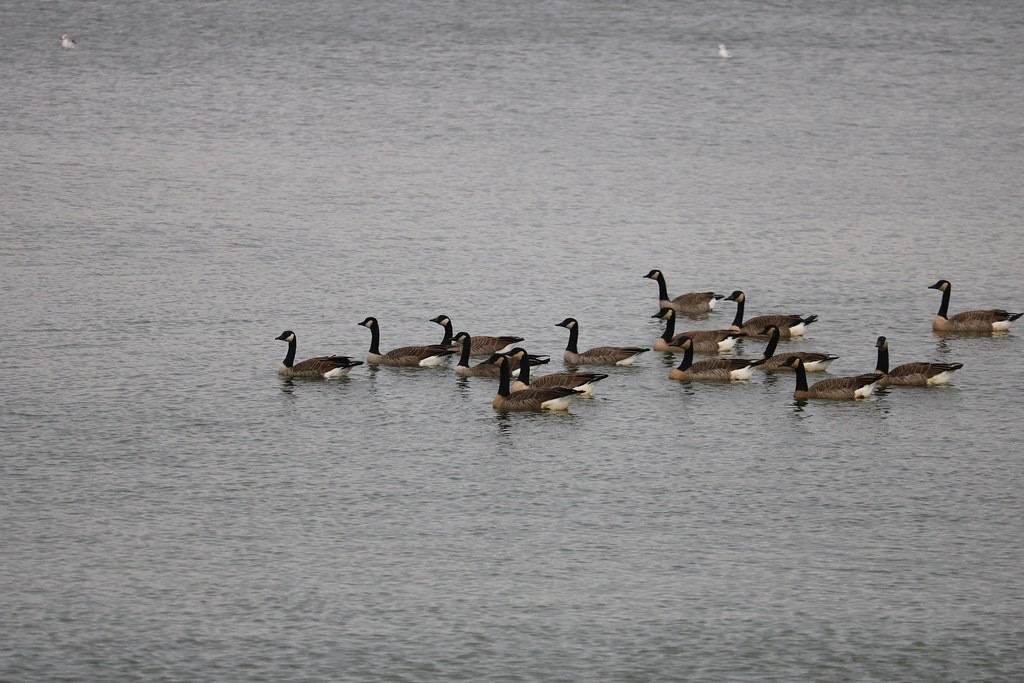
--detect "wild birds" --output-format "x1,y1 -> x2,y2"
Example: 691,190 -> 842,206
716,42 -> 733,59
274,262 -> 1024,414
59,32 -> 78,49
